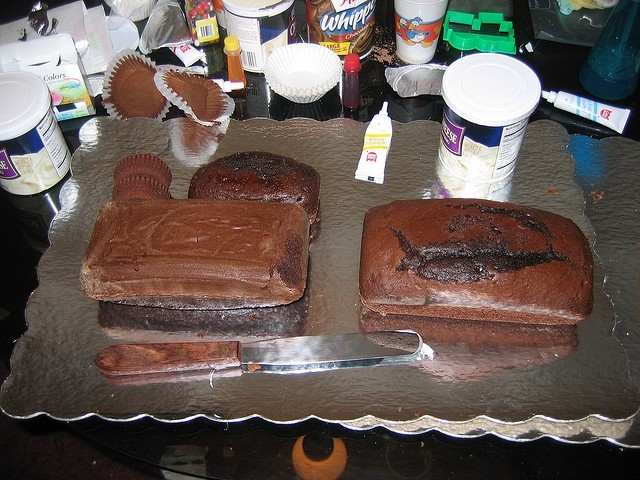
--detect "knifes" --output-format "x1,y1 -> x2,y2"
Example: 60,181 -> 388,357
94,330 -> 421,378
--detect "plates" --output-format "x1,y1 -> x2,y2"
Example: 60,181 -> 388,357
107,16 -> 139,56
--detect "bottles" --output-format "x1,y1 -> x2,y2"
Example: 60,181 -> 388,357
0,33 -> 98,122
306,0 -> 375,64
435,161 -> 511,202
344,54 -> 362,108
223,37 -> 247,89
224,0 -> 295,74
185,1 -> 221,51
394,0 -> 449,64
1,73 -> 71,196
439,52 -> 542,182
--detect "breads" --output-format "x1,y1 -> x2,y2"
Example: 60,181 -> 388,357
113,177 -> 170,201
114,154 -> 172,189
189,151 -> 320,224
359,197 -> 594,324
80,200 -> 311,310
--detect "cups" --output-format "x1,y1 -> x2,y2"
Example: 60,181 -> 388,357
579,1 -> 639,101
